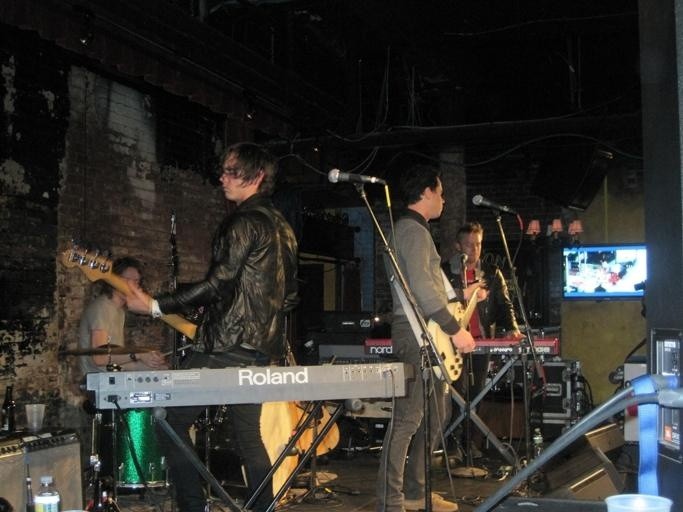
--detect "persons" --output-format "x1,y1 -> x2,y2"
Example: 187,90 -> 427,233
75,252 -> 172,379
440,218 -> 527,471
122,139 -> 305,511
374,159 -> 477,510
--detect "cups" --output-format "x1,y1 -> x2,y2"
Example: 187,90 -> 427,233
605,492 -> 672,511
24,404 -> 44,429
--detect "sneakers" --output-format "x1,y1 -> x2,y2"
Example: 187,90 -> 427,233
471,442 -> 483,459
442,458 -> 460,468
405,492 -> 458,512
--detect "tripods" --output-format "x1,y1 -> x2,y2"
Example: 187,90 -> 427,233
294,405 -> 360,504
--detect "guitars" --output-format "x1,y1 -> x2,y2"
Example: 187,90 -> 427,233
282,334 -> 339,456
428,277 -> 485,383
60,239 -> 198,341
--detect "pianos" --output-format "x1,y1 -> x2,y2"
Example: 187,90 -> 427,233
366,337 -> 558,356
86,364 -> 414,409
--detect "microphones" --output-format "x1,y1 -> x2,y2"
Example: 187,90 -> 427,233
328,169 -> 381,184
472,194 -> 518,216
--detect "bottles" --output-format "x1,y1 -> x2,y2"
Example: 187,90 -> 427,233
533,427 -> 543,457
0,385 -> 16,431
33,476 -> 59,511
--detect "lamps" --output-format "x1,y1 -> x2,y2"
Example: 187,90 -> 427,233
518,216 -> 583,244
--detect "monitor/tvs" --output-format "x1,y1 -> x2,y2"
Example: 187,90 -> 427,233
561,242 -> 647,301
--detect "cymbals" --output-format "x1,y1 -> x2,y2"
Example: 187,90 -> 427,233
59,346 -> 152,354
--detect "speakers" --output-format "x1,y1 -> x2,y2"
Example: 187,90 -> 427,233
300,216 -> 354,260
530,134 -> 613,212
0,431 -> 83,512
